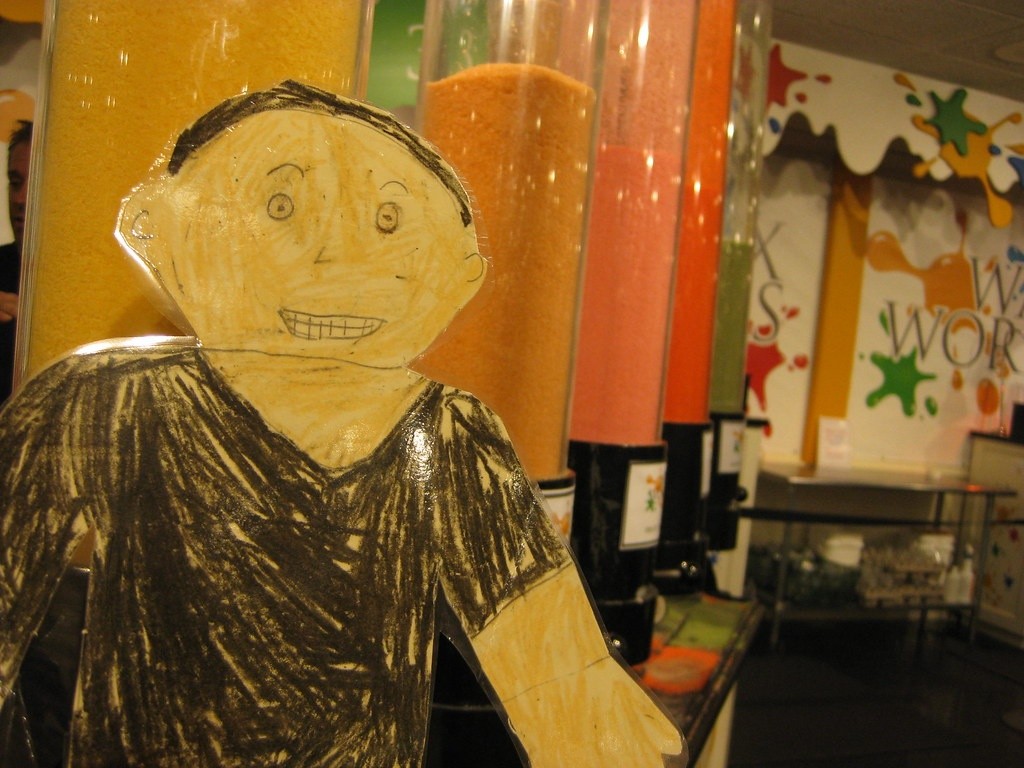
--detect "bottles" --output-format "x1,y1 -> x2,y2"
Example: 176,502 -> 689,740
962,562 -> 975,605
943,566 -> 960,604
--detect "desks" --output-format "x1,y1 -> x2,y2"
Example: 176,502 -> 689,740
759,468 -> 1018,648
